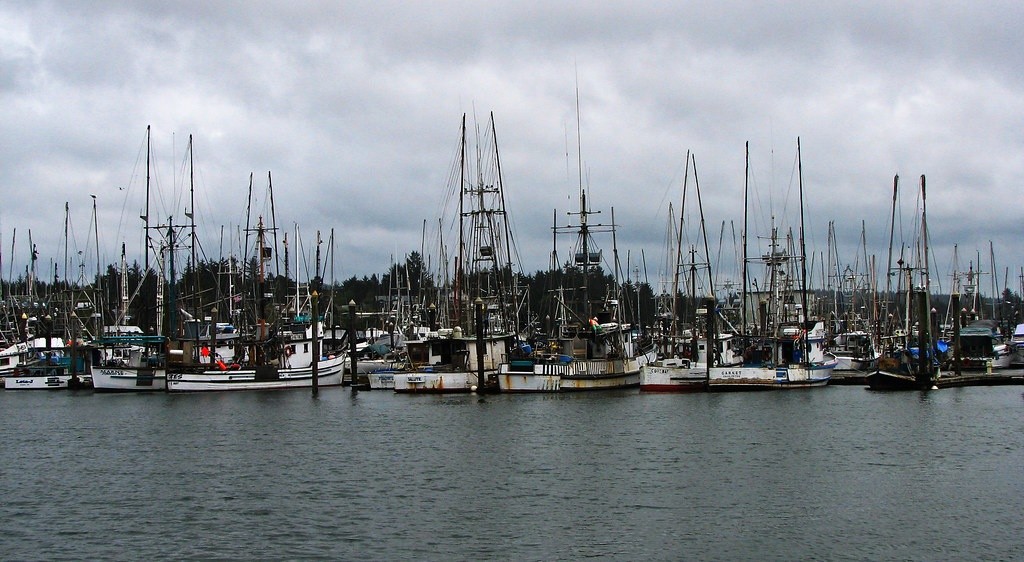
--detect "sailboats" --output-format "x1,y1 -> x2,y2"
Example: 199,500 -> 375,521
1,86 -> 1024,397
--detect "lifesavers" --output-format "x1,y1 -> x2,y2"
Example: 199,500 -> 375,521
285,346 -> 292,357
